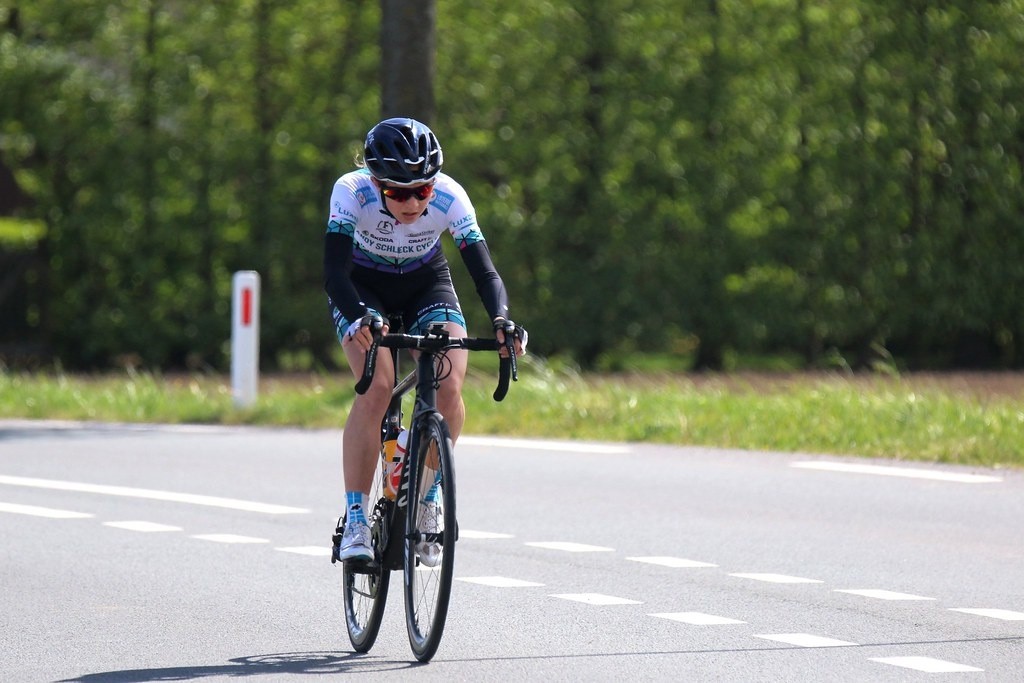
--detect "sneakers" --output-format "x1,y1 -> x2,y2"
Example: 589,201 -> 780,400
414,500 -> 444,567
339,521 -> 375,562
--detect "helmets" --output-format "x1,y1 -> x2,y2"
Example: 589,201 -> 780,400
363,117 -> 443,186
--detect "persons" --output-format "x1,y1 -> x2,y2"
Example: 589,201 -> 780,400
322,118 -> 528,566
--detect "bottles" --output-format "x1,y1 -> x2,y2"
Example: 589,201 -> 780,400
382,427 -> 404,501
387,430 -> 409,494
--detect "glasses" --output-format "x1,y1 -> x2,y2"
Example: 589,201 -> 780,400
378,177 -> 440,202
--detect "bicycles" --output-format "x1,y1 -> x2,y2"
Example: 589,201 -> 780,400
330,313 -> 521,661
